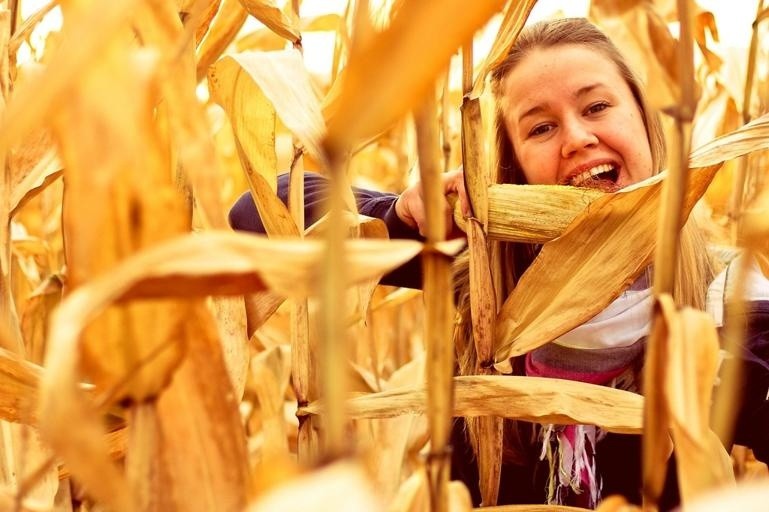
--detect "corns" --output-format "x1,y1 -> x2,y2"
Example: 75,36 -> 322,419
456,183 -> 613,245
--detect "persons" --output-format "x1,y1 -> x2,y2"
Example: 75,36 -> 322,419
227,17 -> 769,512
